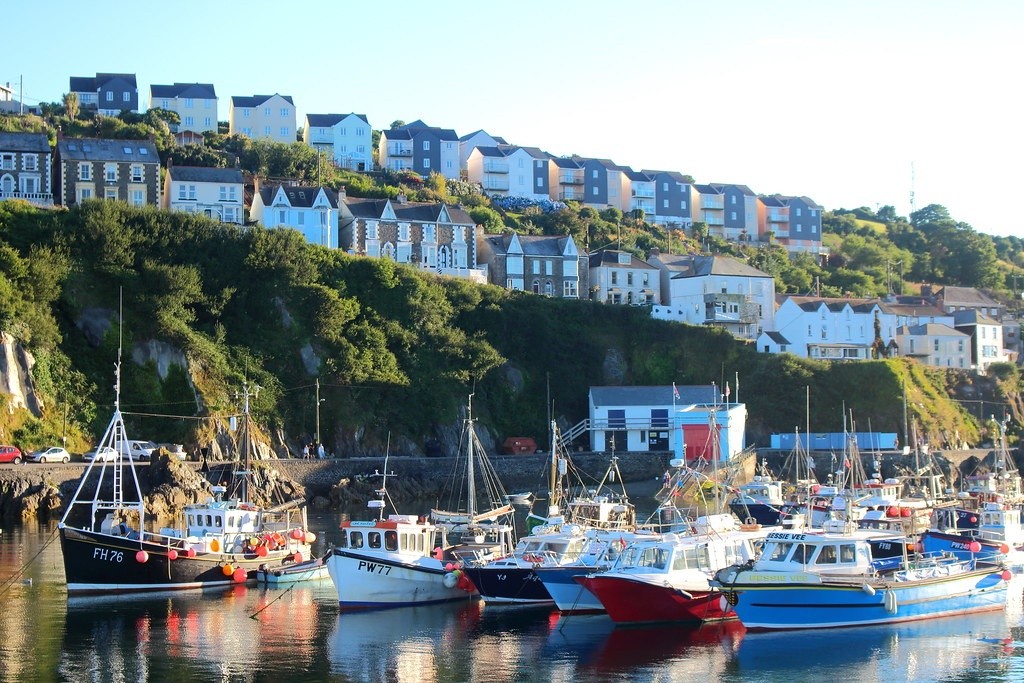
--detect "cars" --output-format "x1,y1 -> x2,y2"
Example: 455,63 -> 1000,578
82,447 -> 118,463
0,445 -> 22,465
25,446 -> 71,464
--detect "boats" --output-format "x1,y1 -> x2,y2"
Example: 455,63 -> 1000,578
503,491 -> 533,500
511,500 -> 532,508
325,390 -> 517,611
57,281 -> 319,606
255,556 -> 330,583
459,373 -> 1024,633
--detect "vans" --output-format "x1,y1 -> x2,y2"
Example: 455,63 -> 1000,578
115,440 -> 156,462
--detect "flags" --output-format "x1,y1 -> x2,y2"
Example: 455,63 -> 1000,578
674,386 -> 680,399
621,537 -> 626,547
726,386 -> 730,395
844,456 -> 851,468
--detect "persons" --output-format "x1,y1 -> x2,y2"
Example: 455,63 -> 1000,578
895,438 -> 899,449
309,442 -> 314,460
318,443 -> 324,459
942,439 -> 950,449
755,463 -> 760,476
663,470 -> 671,489
303,445 -> 309,461
827,478 -> 833,484
957,437 -> 961,449
